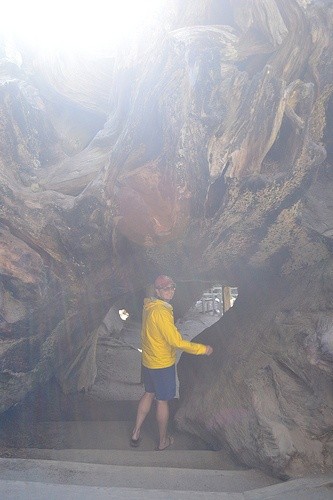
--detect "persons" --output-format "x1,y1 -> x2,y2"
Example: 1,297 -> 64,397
129,275 -> 213,451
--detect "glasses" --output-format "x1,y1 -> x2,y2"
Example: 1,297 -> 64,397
159,287 -> 176,291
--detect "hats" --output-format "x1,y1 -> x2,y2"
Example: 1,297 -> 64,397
154,275 -> 176,288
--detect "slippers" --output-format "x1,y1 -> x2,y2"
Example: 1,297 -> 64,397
130,432 -> 143,447
157,435 -> 175,450
